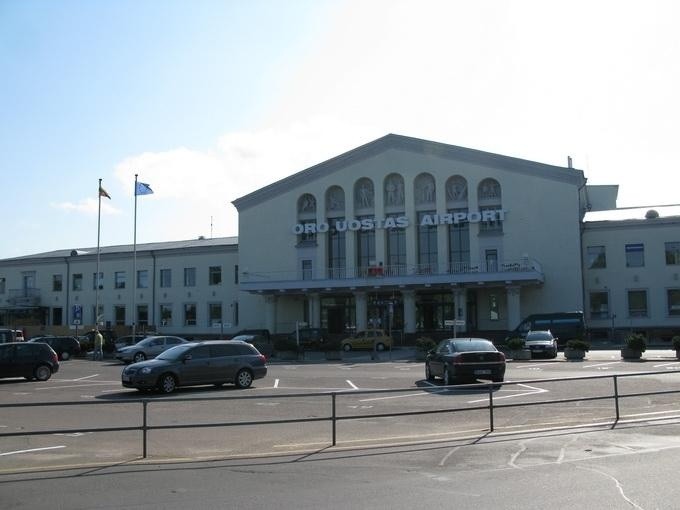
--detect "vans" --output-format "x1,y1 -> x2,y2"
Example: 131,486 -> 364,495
507,312 -> 586,343
0,329 -> 24,344
223,330 -> 270,345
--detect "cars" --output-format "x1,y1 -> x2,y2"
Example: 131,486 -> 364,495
224,336 -> 272,359
124,341 -> 269,390
116,337 -> 188,364
110,334 -> 148,352
424,337 -> 505,388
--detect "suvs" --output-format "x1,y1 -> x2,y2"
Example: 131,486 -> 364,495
524,327 -> 560,360
0,342 -> 60,383
277,328 -> 328,350
79,330 -> 115,350
342,328 -> 391,352
26,336 -> 80,360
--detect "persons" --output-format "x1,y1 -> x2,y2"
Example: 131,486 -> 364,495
91,330 -> 103,360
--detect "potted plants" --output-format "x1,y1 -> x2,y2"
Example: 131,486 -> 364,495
671,334 -> 680,359
620,333 -> 647,360
507,338 -> 532,360
562,339 -> 591,361
415,336 -> 436,360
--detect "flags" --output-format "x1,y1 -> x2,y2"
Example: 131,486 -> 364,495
100,184 -> 111,200
135,181 -> 153,194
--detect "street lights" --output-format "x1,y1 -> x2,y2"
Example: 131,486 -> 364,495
64,250 -> 78,327
149,250 -> 158,332
